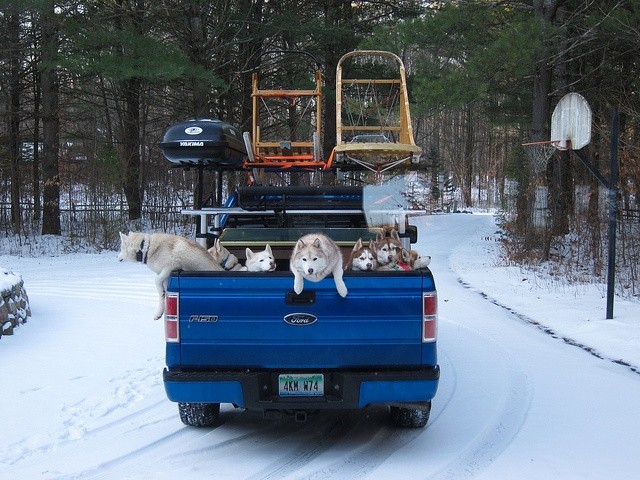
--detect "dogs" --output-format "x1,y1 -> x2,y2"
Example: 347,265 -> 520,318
291,233 -> 348,297
116,231 -> 226,320
372,227 -> 402,267
379,247 -> 431,270
206,238 -> 242,271
238,244 -> 276,271
343,237 -> 377,272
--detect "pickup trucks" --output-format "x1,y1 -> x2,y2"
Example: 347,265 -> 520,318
163,184 -> 441,429
